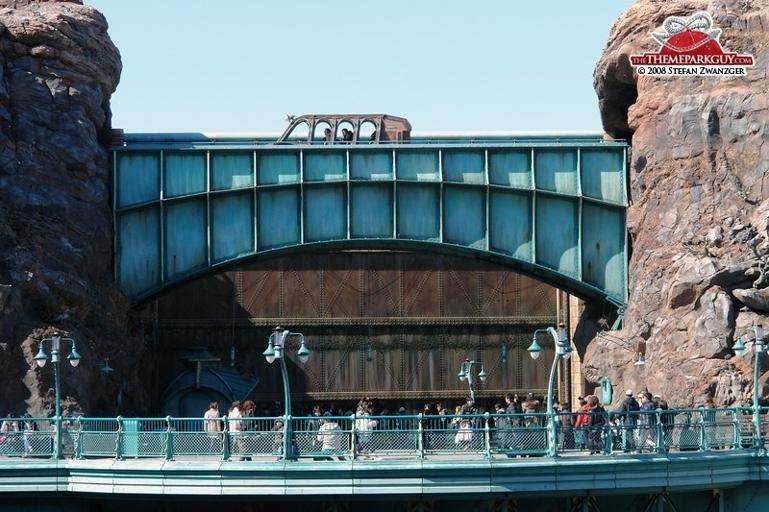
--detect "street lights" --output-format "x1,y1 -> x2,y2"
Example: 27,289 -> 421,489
457,360 -> 488,407
263,326 -> 310,458
33,331 -> 82,460
527,326 -> 573,455
732,325 -> 769,447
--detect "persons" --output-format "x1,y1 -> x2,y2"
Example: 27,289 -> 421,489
0,409 -> 70,459
349,131 -> 353,138
160,421 -> 176,458
203,388 -> 721,462
322,128 -> 331,145
338,128 -> 352,145
368,131 -> 376,144
